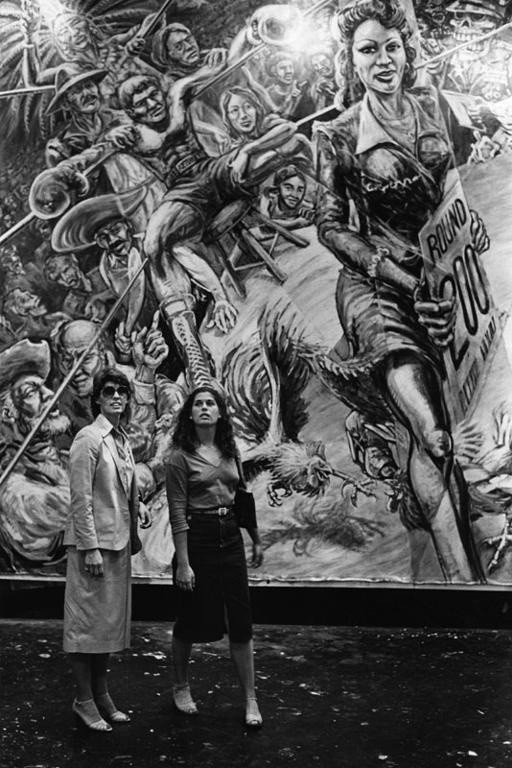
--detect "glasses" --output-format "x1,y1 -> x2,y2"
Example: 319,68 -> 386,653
103,386 -> 127,396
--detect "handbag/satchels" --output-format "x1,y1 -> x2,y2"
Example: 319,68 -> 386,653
234,489 -> 257,528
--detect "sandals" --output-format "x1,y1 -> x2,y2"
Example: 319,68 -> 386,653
95,693 -> 131,722
73,698 -> 112,731
174,685 -> 198,714
245,697 -> 263,726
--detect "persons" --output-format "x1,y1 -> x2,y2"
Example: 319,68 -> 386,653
165,388 -> 264,726
64,368 -> 153,733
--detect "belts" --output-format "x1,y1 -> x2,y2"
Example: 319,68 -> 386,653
189,508 -> 230,516
